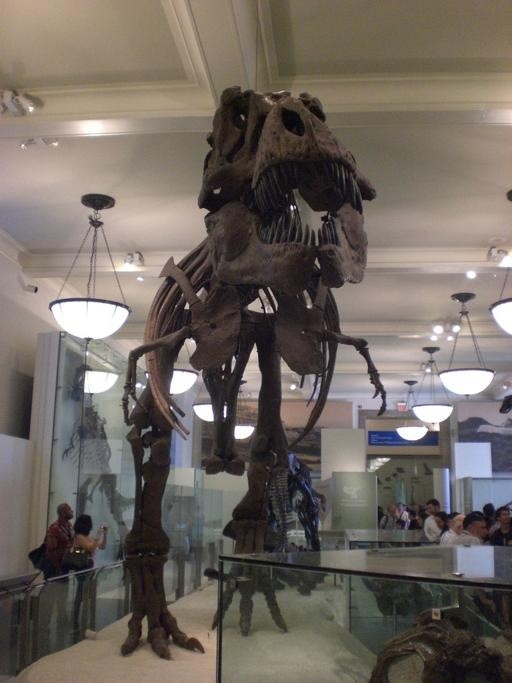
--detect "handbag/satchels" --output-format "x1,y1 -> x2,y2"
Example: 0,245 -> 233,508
65,550 -> 88,568
28,547 -> 49,569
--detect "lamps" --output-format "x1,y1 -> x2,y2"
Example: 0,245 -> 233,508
0,88 -> 39,117
18,137 -> 60,151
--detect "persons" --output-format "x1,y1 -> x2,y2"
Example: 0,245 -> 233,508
360,576 -> 511,639
39,502 -> 75,641
65,513 -> 109,636
377,497 -> 511,546
